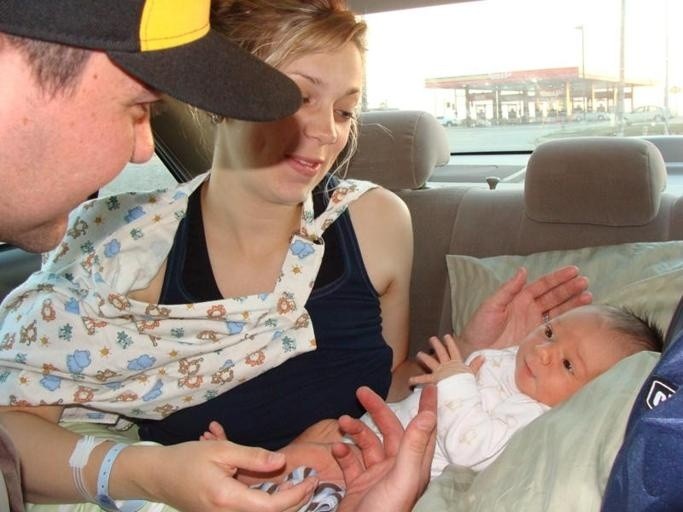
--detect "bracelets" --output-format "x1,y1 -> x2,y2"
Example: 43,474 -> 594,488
96,441 -> 147,512
69,429 -> 111,504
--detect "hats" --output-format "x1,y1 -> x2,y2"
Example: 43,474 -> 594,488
1,1 -> 302,121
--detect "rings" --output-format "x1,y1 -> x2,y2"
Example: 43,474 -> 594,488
543,312 -> 550,320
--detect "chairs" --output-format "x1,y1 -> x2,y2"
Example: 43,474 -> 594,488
344,109 -> 683,358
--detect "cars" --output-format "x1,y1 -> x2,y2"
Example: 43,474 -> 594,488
573,105 -> 672,121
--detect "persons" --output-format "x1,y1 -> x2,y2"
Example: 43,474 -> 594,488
200,302 -> 664,501
1,1 -> 435,512
1,2 -> 593,512
